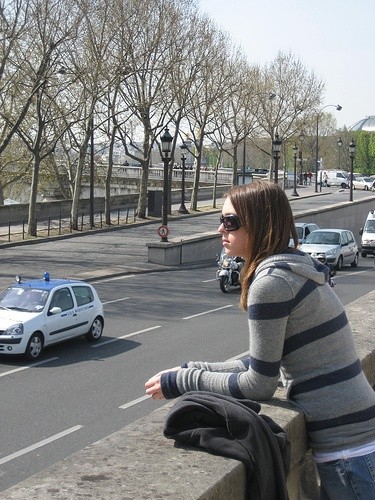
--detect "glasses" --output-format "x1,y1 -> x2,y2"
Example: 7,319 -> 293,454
218,213 -> 253,232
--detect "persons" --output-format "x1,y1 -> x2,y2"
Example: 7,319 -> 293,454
297,169 -> 329,187
144,181 -> 375,499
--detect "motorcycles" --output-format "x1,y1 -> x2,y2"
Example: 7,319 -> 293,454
215,246 -> 246,293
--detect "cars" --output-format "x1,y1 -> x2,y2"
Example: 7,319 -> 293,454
0,271 -> 106,362
288,222 -> 318,249
348,177 -> 374,192
297,227 -> 359,271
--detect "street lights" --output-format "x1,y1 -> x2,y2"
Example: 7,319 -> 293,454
60,68 -> 95,229
337,137 -> 342,169
292,143 -> 300,196
315,104 -> 343,191
177,143 -> 188,213
272,133 -> 281,185
348,137 -> 356,202
297,128 -> 305,185
157,124 -> 174,241
241,91 -> 275,186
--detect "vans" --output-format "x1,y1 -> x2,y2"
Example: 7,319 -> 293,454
358,210 -> 375,259
317,170 -> 349,189
